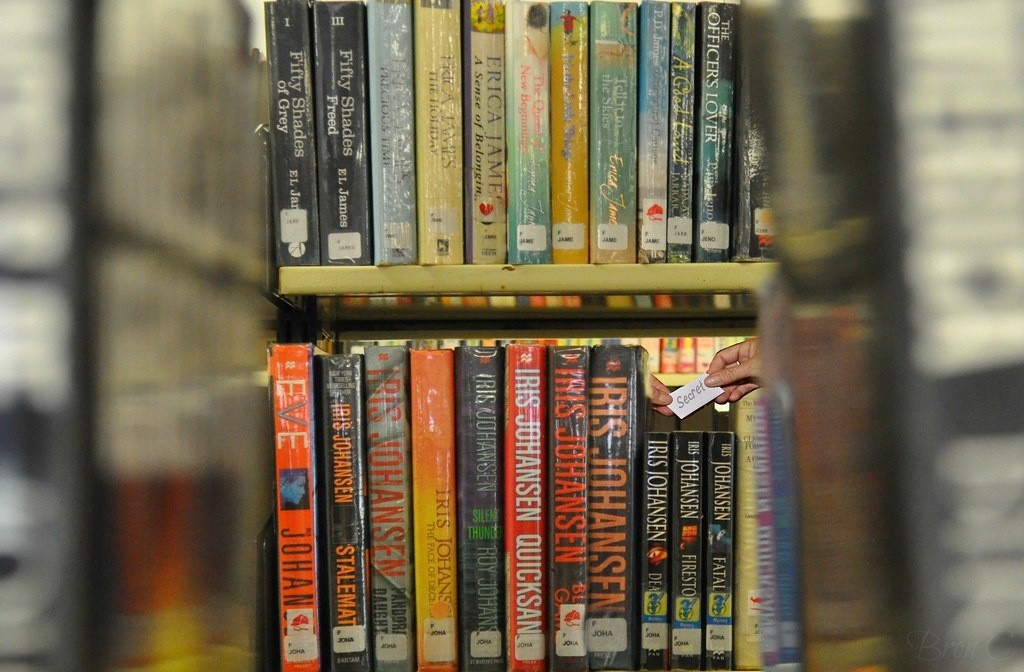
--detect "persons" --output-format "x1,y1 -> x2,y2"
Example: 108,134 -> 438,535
706,338 -> 765,405
649,374 -> 675,417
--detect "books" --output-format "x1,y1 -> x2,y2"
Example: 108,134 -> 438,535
251,2 -> 804,672
